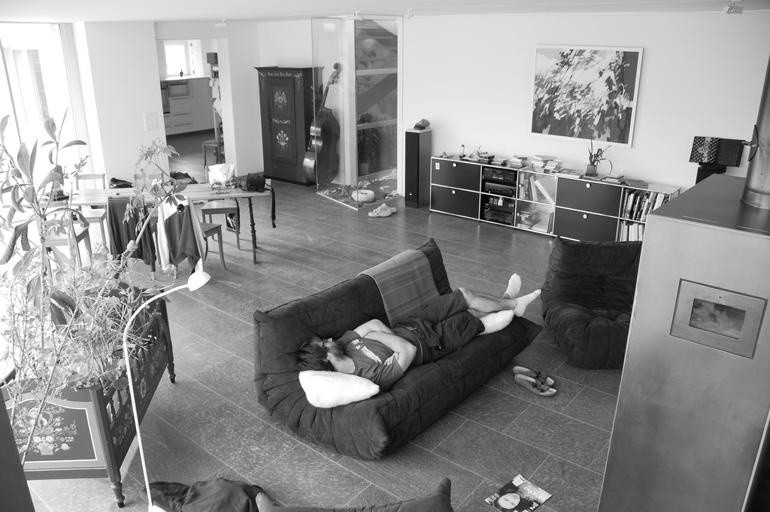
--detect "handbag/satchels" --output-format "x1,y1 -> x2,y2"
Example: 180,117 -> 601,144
247,173 -> 266,193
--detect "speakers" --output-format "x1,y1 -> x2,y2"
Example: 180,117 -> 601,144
404,129 -> 431,209
695,166 -> 726,185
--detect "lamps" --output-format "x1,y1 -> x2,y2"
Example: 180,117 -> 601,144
122,257 -> 212,512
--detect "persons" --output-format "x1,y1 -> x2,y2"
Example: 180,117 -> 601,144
296,269 -> 545,393
493,477 -> 539,511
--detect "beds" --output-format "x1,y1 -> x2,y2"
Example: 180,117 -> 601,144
0,284 -> 177,512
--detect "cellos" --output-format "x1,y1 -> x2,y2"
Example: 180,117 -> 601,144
303,63 -> 339,185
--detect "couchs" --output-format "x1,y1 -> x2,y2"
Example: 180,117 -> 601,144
254,238 -> 542,462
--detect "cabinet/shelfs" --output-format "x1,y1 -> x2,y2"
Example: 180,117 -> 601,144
254,65 -> 324,189
428,153 -> 680,245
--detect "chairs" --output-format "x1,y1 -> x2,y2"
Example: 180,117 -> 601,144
24,164 -> 272,281
202,105 -> 223,169
540,234 -> 644,369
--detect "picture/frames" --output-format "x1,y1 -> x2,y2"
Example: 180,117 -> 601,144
529,45 -> 644,149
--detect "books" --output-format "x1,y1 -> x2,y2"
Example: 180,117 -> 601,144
483,472 -> 554,512
462,147 -> 669,243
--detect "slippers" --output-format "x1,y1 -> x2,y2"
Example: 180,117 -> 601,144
513,366 -> 557,397
368,203 -> 397,217
